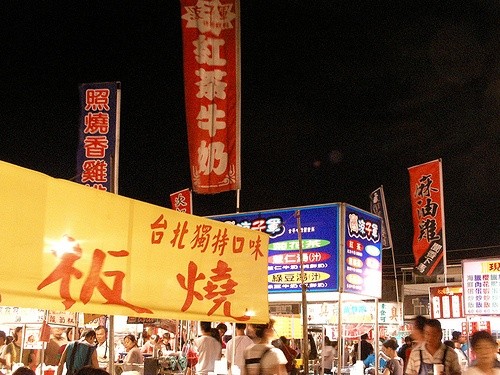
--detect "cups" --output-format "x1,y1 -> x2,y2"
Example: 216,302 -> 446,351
44,370 -> 55,375
294,359 -> 303,369
433,364 -> 444,375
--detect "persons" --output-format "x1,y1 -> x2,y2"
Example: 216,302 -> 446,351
0,314 -> 500,375
